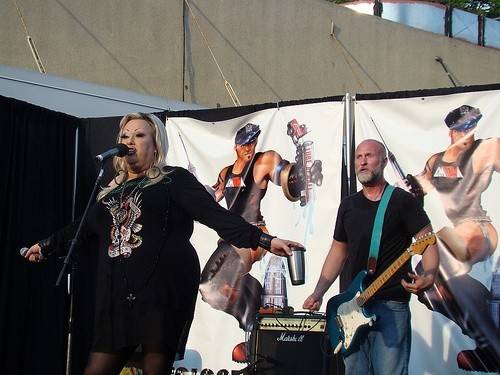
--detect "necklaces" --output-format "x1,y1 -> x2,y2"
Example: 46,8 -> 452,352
120,177 -> 145,209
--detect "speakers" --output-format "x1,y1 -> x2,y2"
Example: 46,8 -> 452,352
253,331 -> 345,375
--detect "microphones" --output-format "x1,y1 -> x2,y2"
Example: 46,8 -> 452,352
20,247 -> 43,261
257,352 -> 277,365
93,143 -> 128,162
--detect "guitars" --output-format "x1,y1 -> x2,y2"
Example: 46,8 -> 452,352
325,231 -> 437,358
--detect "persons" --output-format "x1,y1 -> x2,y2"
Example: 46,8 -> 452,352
25,112 -> 306,375
303,139 -> 440,375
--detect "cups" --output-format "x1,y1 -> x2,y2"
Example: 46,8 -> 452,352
286,247 -> 305,286
